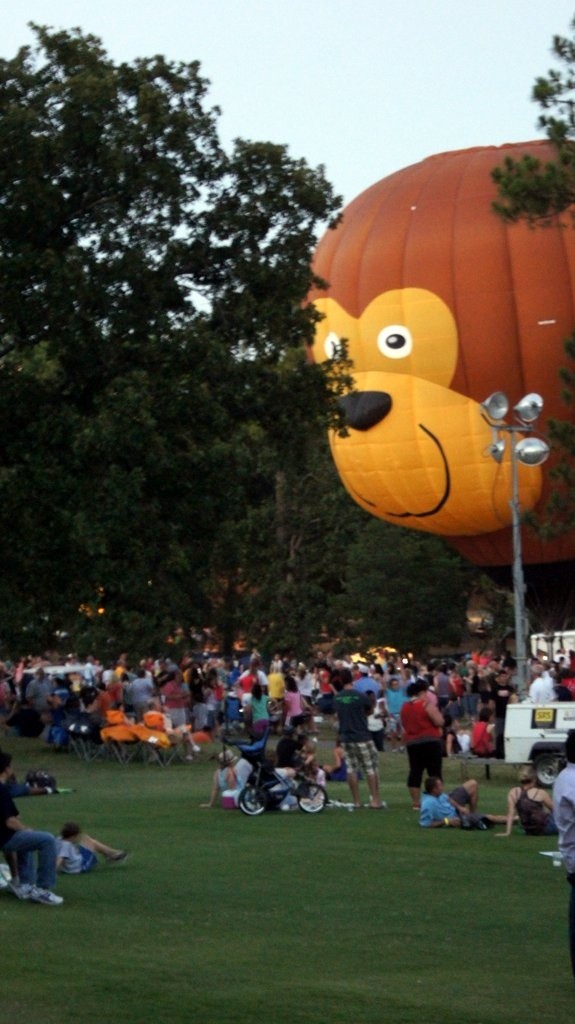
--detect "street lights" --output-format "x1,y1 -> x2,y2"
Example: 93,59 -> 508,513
480,390 -> 550,700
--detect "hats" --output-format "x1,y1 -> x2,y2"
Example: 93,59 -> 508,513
518,765 -> 534,781
219,750 -> 238,764
61,822 -> 80,838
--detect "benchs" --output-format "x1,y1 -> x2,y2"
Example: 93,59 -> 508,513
451,752 -> 505,779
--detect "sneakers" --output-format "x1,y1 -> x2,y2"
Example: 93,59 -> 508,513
31,886 -> 63,905
20,884 -> 36,899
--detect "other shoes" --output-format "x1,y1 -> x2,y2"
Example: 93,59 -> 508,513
108,849 -> 128,862
9,880 -> 22,895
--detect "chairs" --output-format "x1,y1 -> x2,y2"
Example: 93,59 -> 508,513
46,704 -> 204,769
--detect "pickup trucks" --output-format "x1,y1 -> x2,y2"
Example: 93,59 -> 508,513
503,702 -> 575,789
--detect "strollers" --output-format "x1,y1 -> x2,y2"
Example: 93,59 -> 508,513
217,720 -> 328,817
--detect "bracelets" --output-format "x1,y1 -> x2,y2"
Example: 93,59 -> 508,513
444,817 -> 449,826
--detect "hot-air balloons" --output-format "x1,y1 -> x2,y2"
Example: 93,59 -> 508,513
297,137 -> 575,671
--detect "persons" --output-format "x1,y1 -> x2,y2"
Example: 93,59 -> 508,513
495,765 -> 558,837
419,777 -> 520,830
332,668 -> 387,808
201,746 -> 326,810
0,749 -> 63,905
400,684 -> 445,811
0,649 -> 240,765
56,821 -> 126,874
232,649 -> 575,782
552,730 -> 575,980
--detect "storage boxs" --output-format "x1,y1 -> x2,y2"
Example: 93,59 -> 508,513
222,789 -> 240,809
